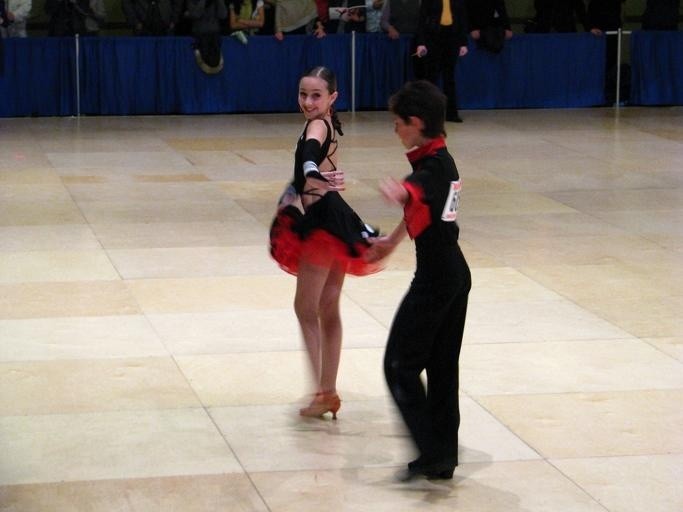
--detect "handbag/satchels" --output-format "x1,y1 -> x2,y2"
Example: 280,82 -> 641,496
192,31 -> 220,56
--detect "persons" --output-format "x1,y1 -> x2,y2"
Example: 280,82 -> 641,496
1,1 -> 682,39
267,64 -> 385,420
412,1 -> 465,123
358,80 -> 473,481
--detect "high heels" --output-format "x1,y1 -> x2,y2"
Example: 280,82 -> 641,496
300,389 -> 341,419
